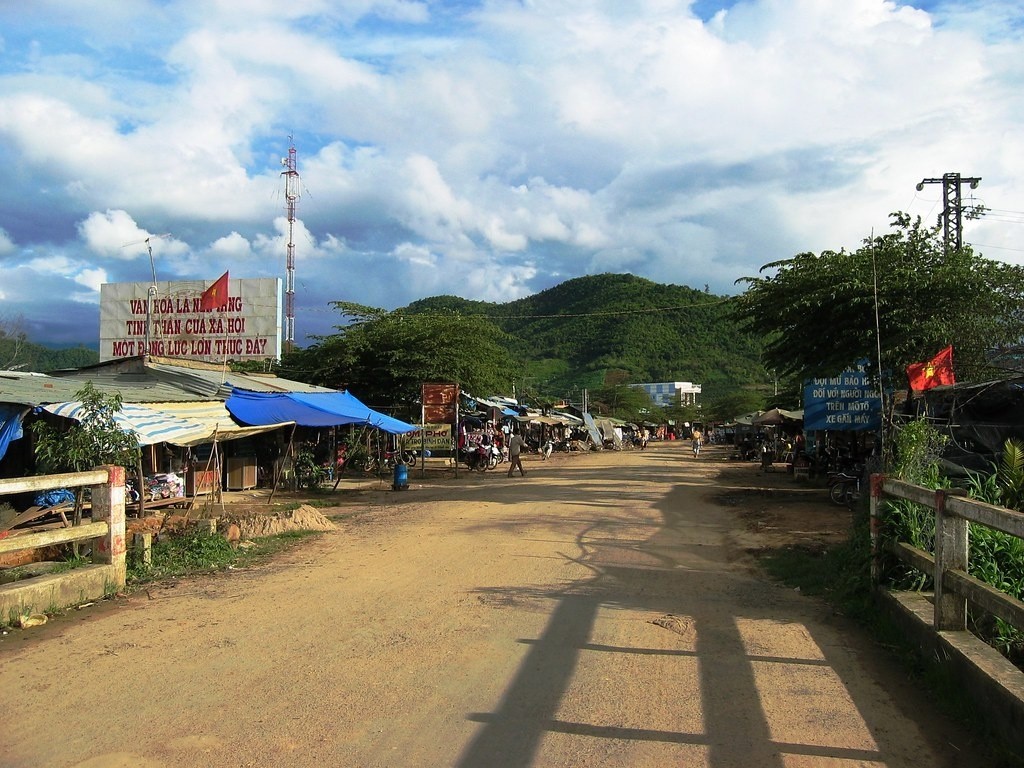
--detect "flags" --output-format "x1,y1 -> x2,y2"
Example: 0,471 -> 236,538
200,272 -> 229,310
905,343 -> 956,392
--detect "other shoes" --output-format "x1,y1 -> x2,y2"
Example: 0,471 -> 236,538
522,471 -> 527,476
508,476 -> 515,478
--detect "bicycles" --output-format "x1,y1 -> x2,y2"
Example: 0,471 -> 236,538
551,442 -> 569,453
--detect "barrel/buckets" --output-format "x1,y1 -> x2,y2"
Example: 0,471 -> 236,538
393,464 -> 407,486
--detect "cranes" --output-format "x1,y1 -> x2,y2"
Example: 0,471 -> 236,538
281,147 -> 301,356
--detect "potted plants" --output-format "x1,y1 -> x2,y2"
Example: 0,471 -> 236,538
321,466 -> 333,482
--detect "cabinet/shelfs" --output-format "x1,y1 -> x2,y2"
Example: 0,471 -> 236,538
187,458 -> 217,496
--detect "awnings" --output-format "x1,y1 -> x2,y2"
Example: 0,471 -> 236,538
33,381 -> 425,519
718,407 -> 803,453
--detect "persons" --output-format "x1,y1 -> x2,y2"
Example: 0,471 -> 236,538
507,428 -> 528,479
788,428 -> 806,475
690,426 -> 702,460
627,426 -> 648,450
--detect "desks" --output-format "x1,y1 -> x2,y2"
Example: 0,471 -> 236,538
227,455 -> 258,492
126,497 -> 196,515
793,466 -> 809,481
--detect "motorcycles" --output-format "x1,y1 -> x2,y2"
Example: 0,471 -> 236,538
465,442 -> 504,471
826,458 -> 866,512
363,451 -> 416,471
